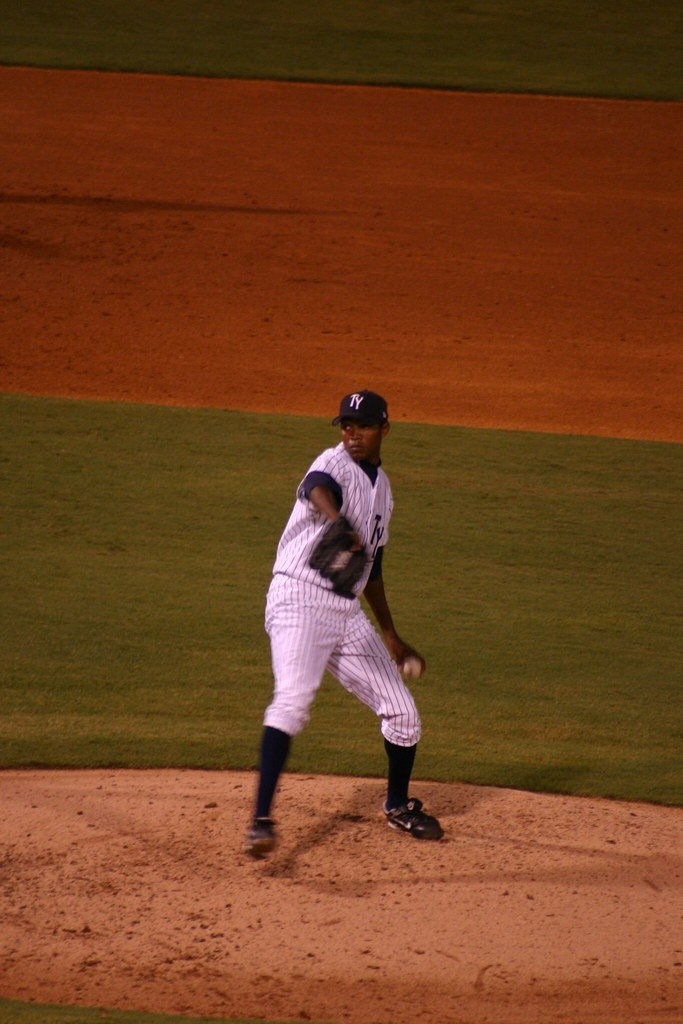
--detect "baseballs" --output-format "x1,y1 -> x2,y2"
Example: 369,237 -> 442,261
403,655 -> 422,677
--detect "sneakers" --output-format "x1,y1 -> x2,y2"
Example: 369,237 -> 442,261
382,797 -> 446,840
246,816 -> 277,859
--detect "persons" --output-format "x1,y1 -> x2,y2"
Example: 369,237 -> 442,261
242,389 -> 444,860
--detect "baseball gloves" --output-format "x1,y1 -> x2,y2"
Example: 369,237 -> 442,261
308,511 -> 368,598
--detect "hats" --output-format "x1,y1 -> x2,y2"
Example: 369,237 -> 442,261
331,389 -> 388,427
